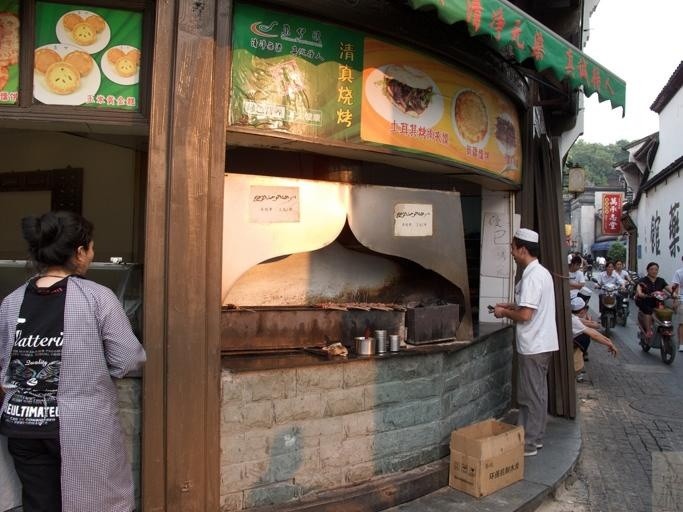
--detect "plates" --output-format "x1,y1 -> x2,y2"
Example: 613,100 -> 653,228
366,63 -> 518,158
33,10 -> 142,106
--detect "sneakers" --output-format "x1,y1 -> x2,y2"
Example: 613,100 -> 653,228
524,445 -> 543,456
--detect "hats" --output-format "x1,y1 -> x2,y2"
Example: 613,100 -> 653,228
571,297 -> 585,311
515,228 -> 539,243
578,286 -> 592,296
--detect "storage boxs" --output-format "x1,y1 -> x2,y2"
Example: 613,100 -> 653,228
448,419 -> 525,499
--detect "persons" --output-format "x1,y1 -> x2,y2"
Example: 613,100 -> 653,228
567,249 -> 636,378
670,255 -> 683,352
633,262 -> 681,338
488,227 -> 560,457
0,209 -> 146,511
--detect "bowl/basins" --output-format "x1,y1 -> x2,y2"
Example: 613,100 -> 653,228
354,336 -> 377,355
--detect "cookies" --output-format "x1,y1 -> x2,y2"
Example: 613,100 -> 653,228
62,13 -> 106,45
107,48 -> 141,77
34,48 -> 94,95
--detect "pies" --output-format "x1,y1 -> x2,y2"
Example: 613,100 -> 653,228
383,65 -> 434,118
0,12 -> 20,91
455,91 -> 488,142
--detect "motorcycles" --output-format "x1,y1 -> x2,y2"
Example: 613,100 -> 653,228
592,276 -> 641,337
637,282 -> 680,364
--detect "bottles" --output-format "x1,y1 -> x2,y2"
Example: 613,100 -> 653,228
374,327 -> 399,353
